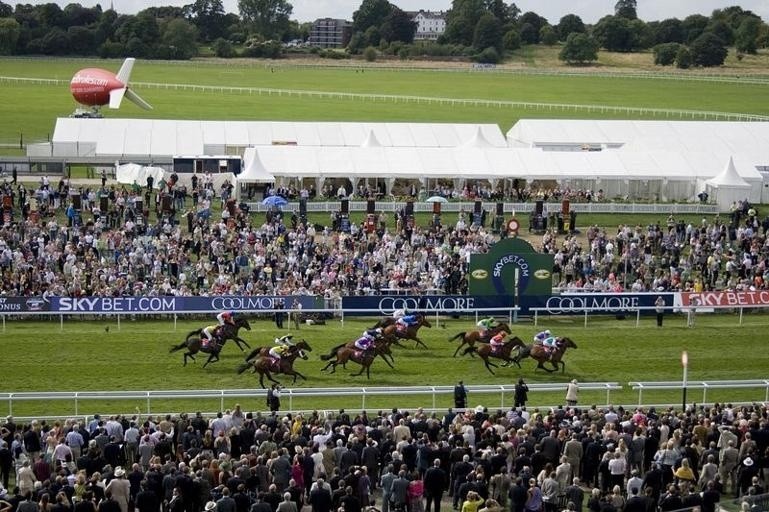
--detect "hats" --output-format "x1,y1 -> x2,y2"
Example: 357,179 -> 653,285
544,329 -> 551,336
203,501 -> 217,511
34,480 -> 42,489
741,457 -> 756,467
489,316 -> 496,324
88,439 -> 97,450
112,464 -> 126,478
498,330 -> 507,338
474,404 -> 483,414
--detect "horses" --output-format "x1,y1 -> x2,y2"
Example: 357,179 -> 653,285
460,336 -> 527,376
237,340 -> 314,389
500,337 -> 579,374
318,312 -> 429,380
166,317 -> 251,368
447,323 -> 513,359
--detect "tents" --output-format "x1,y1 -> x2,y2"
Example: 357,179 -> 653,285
705,157 -> 752,210
235,148 -> 276,202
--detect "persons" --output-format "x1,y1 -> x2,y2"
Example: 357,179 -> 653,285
201,324 -> 222,347
268,345 -> 290,367
266,383 -> 281,413
355,336 -> 378,359
542,336 -> 562,361
291,295 -> 303,331
396,314 -> 417,330
274,333 -> 296,354
217,309 -> 236,332
686,292 -> 697,330
565,378 -> 581,408
418,294 -> 427,323
476,315 -> 502,337
454,379 -> 469,414
653,294 -> 667,330
489,331 -> 508,355
533,329 -> 552,343
1,401 -> 768,512
362,328 -> 385,342
273,297 -> 285,329
509,178 -> 769,317
0,166 -> 509,321
512,378 -> 530,409
